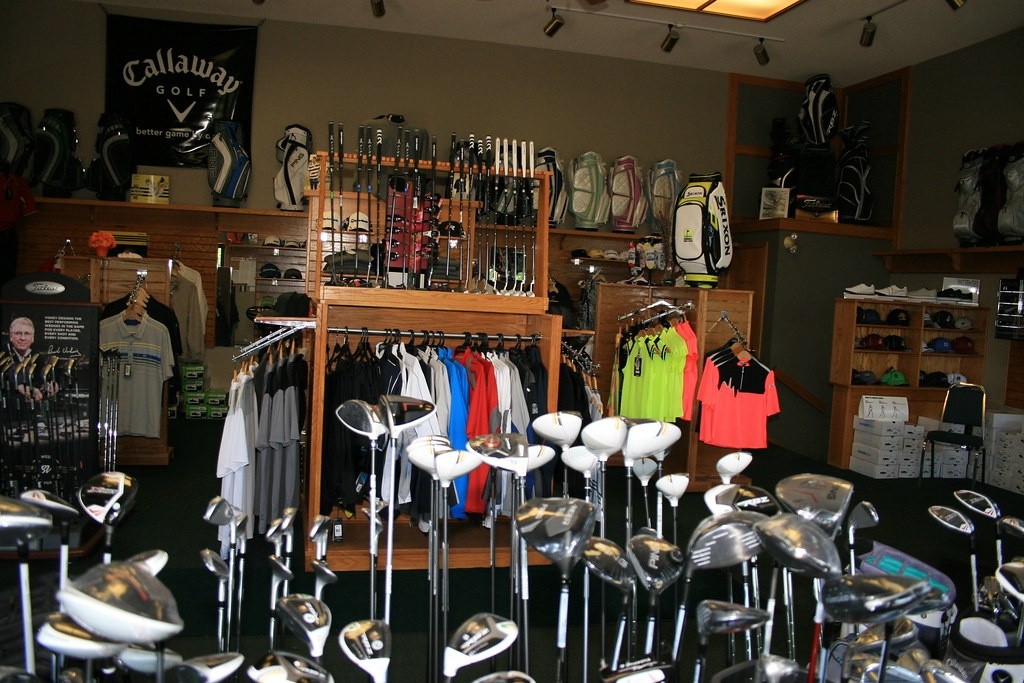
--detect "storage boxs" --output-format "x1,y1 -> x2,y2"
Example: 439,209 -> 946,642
985,405 -> 1024,498
182,365 -> 228,422
848,416 -> 983,483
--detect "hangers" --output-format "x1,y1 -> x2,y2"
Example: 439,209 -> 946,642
232,326 -> 297,382
559,339 -> 604,417
124,280 -> 150,324
170,255 -> 181,280
327,325 -> 539,374
730,330 -> 752,366
614,305 -> 690,358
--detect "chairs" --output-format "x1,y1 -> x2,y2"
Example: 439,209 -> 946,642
918,382 -> 987,492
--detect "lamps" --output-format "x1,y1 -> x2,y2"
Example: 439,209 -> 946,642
252,0 -> 263,6
543,9 -> 564,37
752,38 -> 770,66
660,23 -> 680,53
859,16 -> 876,49
946,0 -> 966,9
370,0 -> 385,18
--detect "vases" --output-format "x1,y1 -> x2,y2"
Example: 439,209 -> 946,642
94,248 -> 109,258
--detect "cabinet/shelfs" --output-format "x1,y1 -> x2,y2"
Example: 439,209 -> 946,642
594,279 -> 755,492
227,242 -> 307,282
89,257 -> 175,467
255,149 -> 597,573
827,385 -> 982,474
828,291 -> 990,389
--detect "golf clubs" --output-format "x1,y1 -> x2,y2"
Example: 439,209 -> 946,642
526,141 -> 535,297
380,393 -> 437,626
510,139 -> 518,296
337,122 -> 349,287
324,119 -> 339,286
335,399 -> 389,622
492,136 -> 501,295
343,125 -> 492,295
518,140 -> 527,296
500,137 -> 510,296
1,408 -> 1024,683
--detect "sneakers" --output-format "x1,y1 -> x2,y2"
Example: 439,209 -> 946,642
845,283 -> 875,295
907,287 -> 937,298
875,284 -> 908,297
937,288 -> 973,302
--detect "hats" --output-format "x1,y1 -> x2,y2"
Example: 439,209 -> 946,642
924,310 -> 978,331
440,219 -> 461,236
919,370 -> 968,386
921,337 -> 975,353
852,367 -> 909,386
856,306 -> 911,326
262,235 -> 307,248
854,334 -> 912,352
284,269 -> 302,279
259,263 -> 281,278
322,211 -> 370,232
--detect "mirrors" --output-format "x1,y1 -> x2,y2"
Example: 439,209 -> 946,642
216,243 -> 259,346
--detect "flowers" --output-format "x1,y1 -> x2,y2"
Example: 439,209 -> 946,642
87,231 -> 117,250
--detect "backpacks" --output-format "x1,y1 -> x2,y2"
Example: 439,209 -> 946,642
766,73 -> 876,225
952,141 -> 1024,244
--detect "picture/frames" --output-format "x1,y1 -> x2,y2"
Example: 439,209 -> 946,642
758,186 -> 790,219
